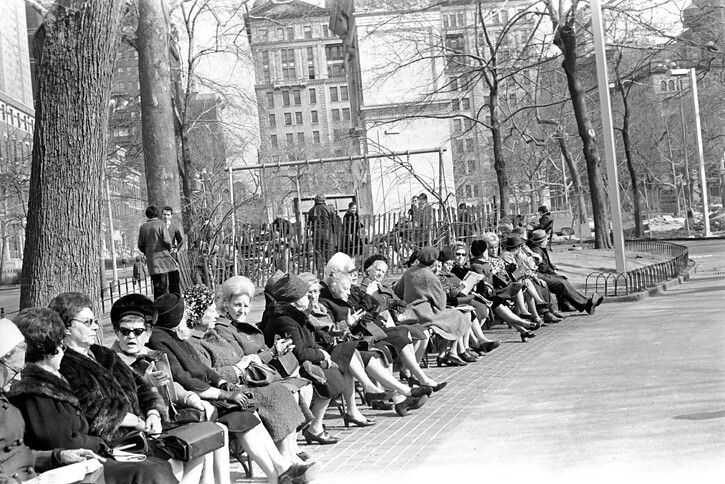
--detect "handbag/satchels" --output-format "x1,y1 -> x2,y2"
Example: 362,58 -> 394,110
148,421 -> 225,463
171,408 -> 218,423
270,334 -> 299,377
110,430 -> 148,454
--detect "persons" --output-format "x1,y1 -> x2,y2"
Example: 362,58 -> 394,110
0,193 -> 604,484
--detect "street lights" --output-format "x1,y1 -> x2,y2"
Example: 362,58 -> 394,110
665,68 -> 711,236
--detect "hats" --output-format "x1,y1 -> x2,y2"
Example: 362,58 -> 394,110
267,269 -> 285,285
438,249 -> 455,261
530,229 -> 547,243
269,273 -> 311,302
471,240 -> 487,256
154,293 -> 184,328
538,206 -> 546,211
417,247 -> 439,266
0,318 -> 24,360
364,254 -> 387,270
505,233 -> 525,247
111,293 -> 158,327
182,284 -> 214,329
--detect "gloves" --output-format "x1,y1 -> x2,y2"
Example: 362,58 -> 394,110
219,389 -> 251,412
218,382 -> 254,399
310,264 -> 517,346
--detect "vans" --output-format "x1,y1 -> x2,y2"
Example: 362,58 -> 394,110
525,209 -> 576,236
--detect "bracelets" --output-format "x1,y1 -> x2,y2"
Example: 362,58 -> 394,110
135,415 -> 141,429
234,364 -> 244,375
270,351 -> 275,357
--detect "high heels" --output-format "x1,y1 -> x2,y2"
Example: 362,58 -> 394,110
263,291 -> 604,483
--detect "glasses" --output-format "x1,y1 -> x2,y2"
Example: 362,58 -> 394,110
119,327 -> 148,336
347,267 -> 361,274
0,360 -> 27,376
455,253 -> 467,256
69,318 -> 98,327
